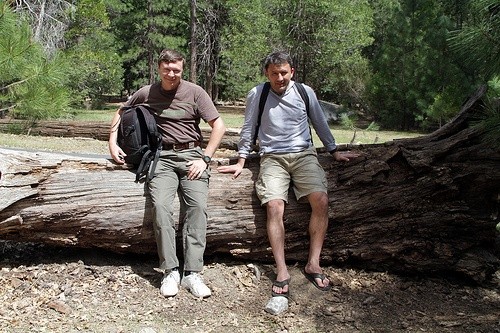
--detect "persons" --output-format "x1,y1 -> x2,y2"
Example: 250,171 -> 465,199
109,50 -> 227,298
217,51 -> 361,297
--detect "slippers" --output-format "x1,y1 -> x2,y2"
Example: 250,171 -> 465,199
303,267 -> 332,291
272,276 -> 291,298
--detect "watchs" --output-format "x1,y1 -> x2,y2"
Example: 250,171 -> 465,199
201,155 -> 211,165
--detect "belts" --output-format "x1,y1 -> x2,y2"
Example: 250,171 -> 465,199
161,141 -> 199,151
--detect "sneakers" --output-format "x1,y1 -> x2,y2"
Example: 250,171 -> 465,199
181,272 -> 211,299
160,268 -> 181,297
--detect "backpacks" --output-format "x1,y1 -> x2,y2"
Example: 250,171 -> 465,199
117,104 -> 162,185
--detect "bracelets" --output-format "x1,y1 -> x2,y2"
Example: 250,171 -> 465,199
330,148 -> 337,154
